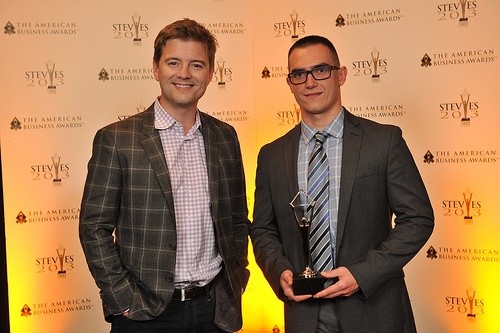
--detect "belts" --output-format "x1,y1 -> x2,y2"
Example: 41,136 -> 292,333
172,283 -> 214,301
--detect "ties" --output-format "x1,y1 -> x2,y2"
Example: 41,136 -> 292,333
307,132 -> 334,288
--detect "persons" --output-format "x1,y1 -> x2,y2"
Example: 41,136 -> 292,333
79,18 -> 251,333
251,35 -> 435,333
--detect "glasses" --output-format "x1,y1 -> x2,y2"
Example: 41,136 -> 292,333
288,66 -> 340,85
